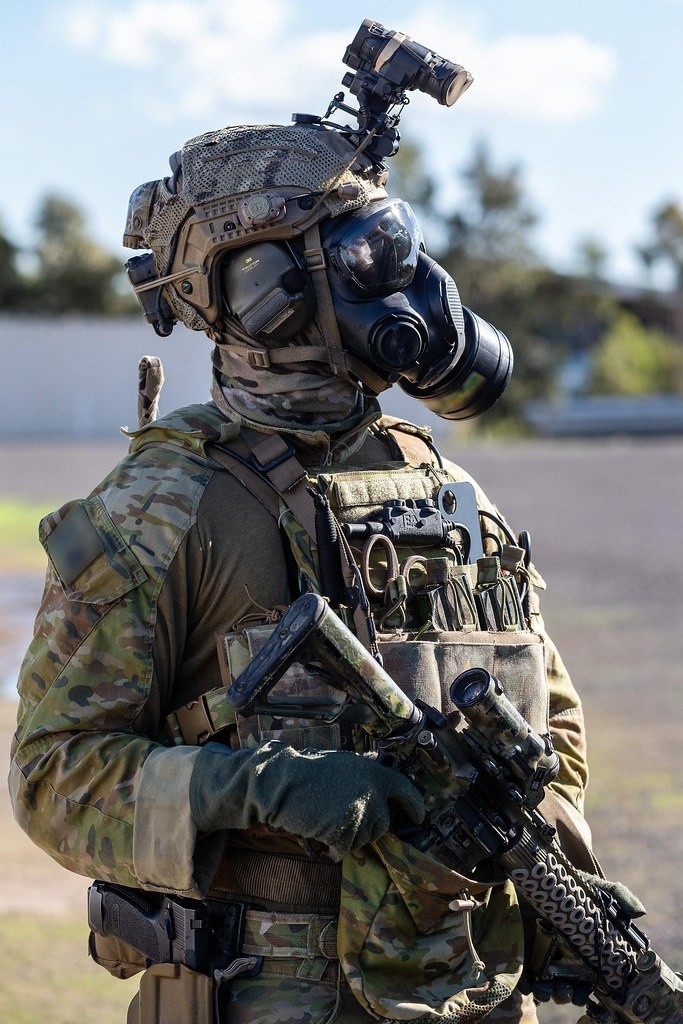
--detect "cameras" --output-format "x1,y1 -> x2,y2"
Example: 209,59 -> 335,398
343,20 -> 468,108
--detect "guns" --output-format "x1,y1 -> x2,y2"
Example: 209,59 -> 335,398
83,882 -> 213,976
225,591 -> 683,1023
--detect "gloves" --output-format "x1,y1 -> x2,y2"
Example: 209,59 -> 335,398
188,743 -> 425,856
518,871 -> 647,1007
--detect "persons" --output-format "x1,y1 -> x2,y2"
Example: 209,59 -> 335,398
6,121 -> 647,1023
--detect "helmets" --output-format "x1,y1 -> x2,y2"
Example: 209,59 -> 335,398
121,122 -> 389,343
